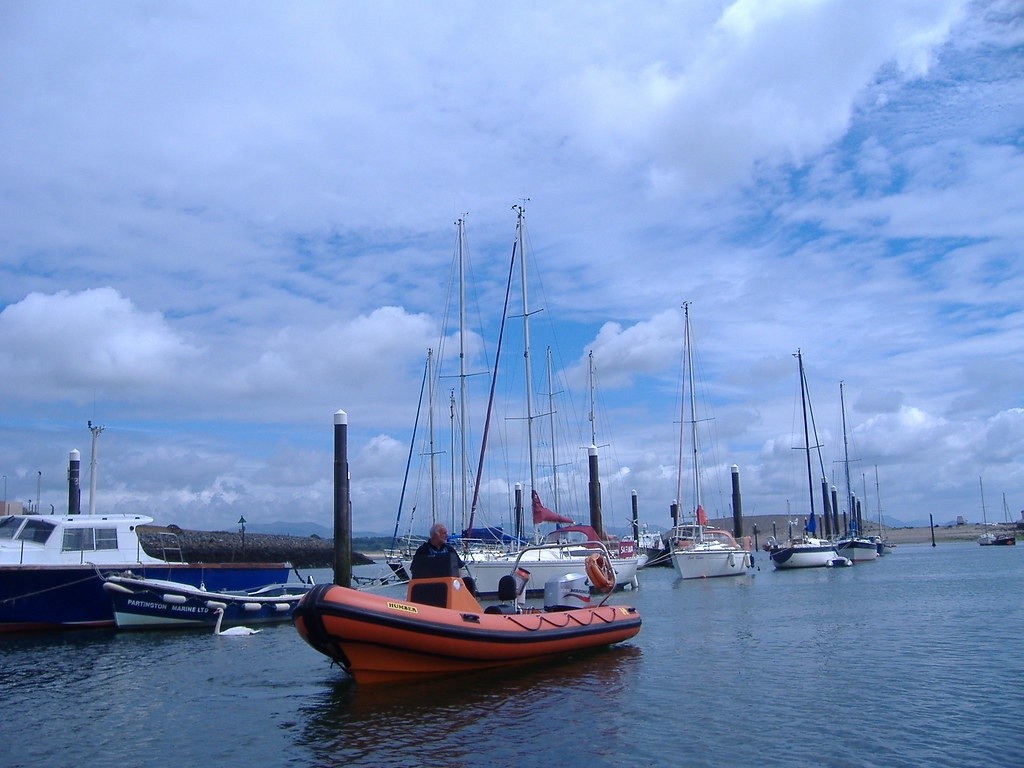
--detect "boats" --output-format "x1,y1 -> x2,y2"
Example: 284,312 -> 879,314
0,511 -> 295,621
104,570 -> 314,630
294,540 -> 642,682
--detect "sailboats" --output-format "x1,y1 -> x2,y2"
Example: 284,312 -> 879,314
874,464 -> 893,547
667,300 -> 751,580
353,202 -> 671,593
833,379 -> 879,561
991,492 -> 1015,545
861,473 -> 883,553
977,476 -> 996,545
771,347 -> 835,568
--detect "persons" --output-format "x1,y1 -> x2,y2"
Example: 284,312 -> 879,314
410,523 -> 466,608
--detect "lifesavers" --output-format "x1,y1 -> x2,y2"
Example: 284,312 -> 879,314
585,553 -> 615,593
